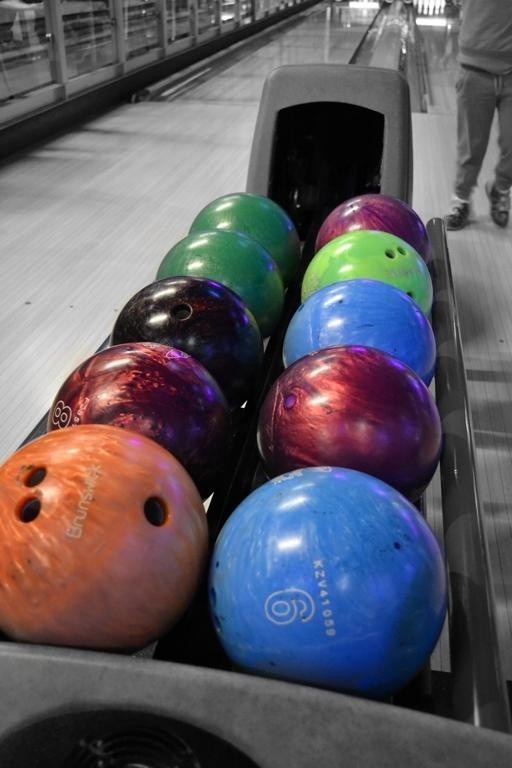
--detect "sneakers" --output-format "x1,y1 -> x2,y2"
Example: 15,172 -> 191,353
443,196 -> 470,232
485,178 -> 512,227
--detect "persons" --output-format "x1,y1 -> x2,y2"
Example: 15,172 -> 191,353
444,0 -> 512,230
